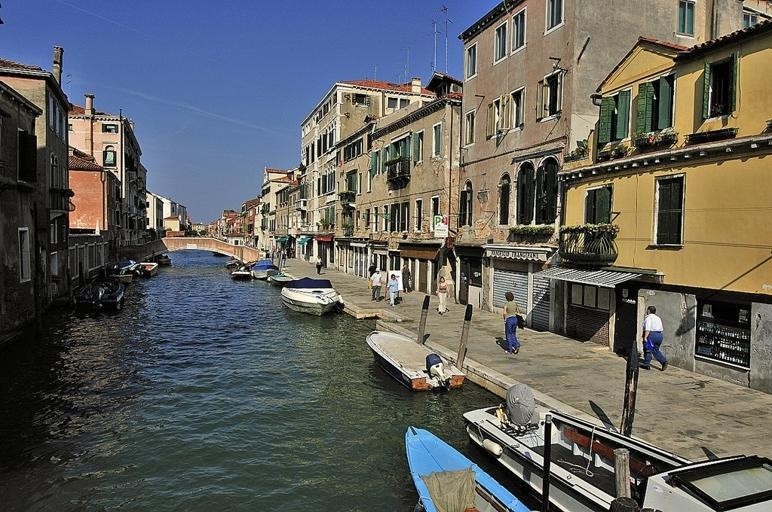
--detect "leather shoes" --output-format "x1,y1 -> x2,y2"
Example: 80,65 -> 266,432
640,361 -> 668,370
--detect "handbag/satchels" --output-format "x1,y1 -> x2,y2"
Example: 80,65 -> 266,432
516,314 -> 527,330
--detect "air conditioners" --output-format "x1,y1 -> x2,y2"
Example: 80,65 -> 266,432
450,83 -> 464,94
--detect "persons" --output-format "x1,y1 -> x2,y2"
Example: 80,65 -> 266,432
502,291 -> 522,355
640,305 -> 668,371
368,263 -> 376,277
386,274 -> 398,306
435,276 -> 450,315
370,267 -> 382,302
315,255 -> 323,273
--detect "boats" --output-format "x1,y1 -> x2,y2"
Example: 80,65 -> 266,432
404,423 -> 534,512
75,253 -> 172,315
280,276 -> 339,318
365,327 -> 467,395
459,398 -> 772,511
226,255 -> 292,288
